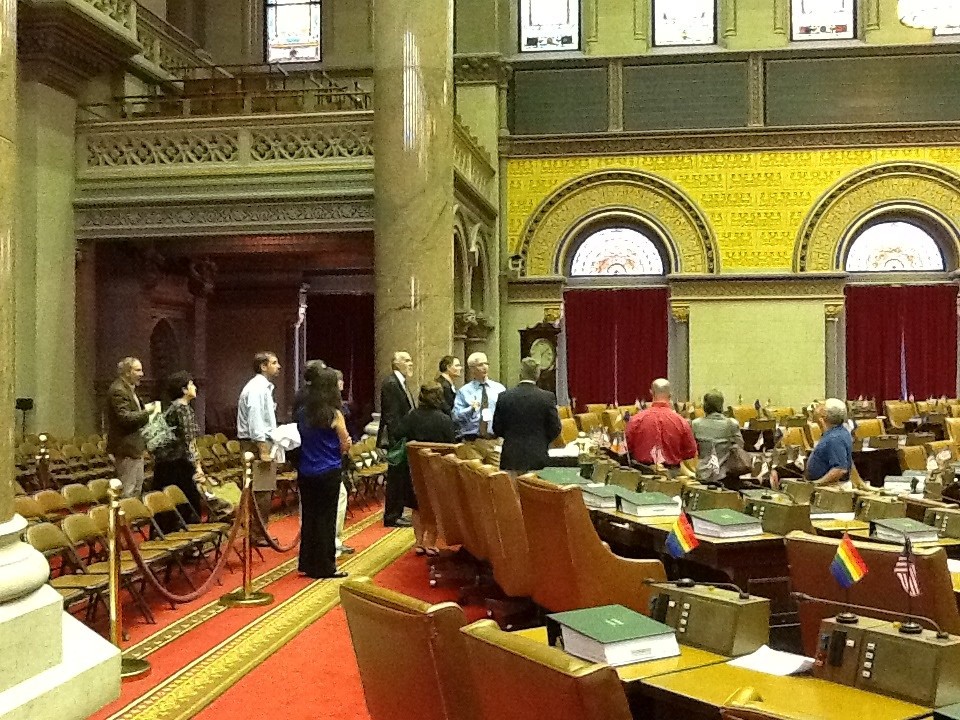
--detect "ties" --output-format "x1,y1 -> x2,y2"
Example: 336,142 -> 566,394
404,380 -> 417,410
479,383 -> 489,438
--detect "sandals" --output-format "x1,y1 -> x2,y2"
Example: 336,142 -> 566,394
415,546 -> 426,556
426,547 -> 444,557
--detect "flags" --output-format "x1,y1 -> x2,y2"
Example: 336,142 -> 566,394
829,529 -> 869,590
664,509 -> 700,560
895,539 -> 925,598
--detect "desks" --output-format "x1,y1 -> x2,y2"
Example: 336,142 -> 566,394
596,508 -> 787,598
847,433 -> 906,485
739,487 -> 871,540
844,528 -> 960,560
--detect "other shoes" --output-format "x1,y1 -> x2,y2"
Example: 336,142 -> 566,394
256,537 -> 279,547
337,544 -> 355,553
183,550 -> 210,558
309,572 -> 348,578
385,516 -> 412,526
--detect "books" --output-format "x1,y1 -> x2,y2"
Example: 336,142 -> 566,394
544,600 -> 684,668
524,408 -> 960,635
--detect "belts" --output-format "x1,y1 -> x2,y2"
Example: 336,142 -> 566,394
640,461 -> 681,470
240,437 -> 272,442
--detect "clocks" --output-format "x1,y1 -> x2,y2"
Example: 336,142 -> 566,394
517,321 -> 562,395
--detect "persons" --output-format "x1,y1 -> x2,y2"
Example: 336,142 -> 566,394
237,349 -> 284,548
432,352 -> 507,468
402,381 -> 459,557
492,356 -> 561,476
291,357 -> 353,580
624,378 -> 696,471
801,396 -> 854,509
687,390 -> 745,493
107,356 -> 156,501
148,371 -> 213,559
376,350 -> 424,528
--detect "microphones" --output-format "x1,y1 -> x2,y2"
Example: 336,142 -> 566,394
744,480 -> 797,503
631,459 -> 672,481
689,476 -> 725,491
642,578 -> 749,600
793,592 -> 950,637
779,465 -> 806,482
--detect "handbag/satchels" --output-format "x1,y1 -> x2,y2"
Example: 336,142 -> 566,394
138,404 -> 178,454
696,447 -> 720,482
729,444 -> 753,475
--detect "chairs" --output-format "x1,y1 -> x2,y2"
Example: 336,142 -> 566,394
13,397 -> 960,720
